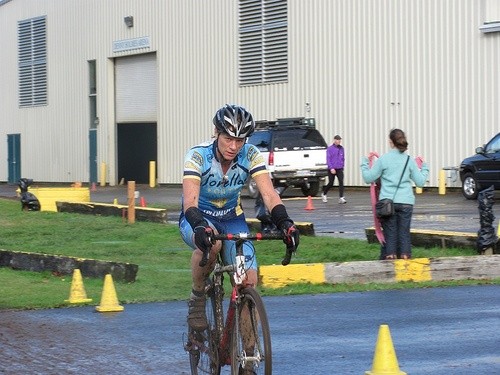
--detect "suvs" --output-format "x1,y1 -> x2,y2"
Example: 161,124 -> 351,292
459,130 -> 500,201
244,119 -> 330,199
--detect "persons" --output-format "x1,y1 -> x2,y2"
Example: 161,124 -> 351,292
321,135 -> 347,203
179,104 -> 300,375
361,129 -> 429,260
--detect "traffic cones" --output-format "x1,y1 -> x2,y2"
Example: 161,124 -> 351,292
94,272 -> 126,313
63,267 -> 92,307
304,196 -> 317,210
363,324 -> 415,375
140,195 -> 147,207
90,182 -> 97,192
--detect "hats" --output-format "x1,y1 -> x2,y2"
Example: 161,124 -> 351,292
334,135 -> 343,140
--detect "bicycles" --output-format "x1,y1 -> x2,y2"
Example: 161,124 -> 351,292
182,232 -> 291,375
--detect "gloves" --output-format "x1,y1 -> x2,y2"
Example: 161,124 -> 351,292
194,220 -> 215,251
283,223 -> 299,250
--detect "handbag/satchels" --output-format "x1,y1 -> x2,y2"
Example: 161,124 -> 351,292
375,198 -> 394,217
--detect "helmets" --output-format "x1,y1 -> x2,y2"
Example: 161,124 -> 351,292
213,104 -> 256,138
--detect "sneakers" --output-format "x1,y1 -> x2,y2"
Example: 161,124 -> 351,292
321,192 -> 328,203
238,363 -> 257,375
338,197 -> 347,204
187,293 -> 209,332
